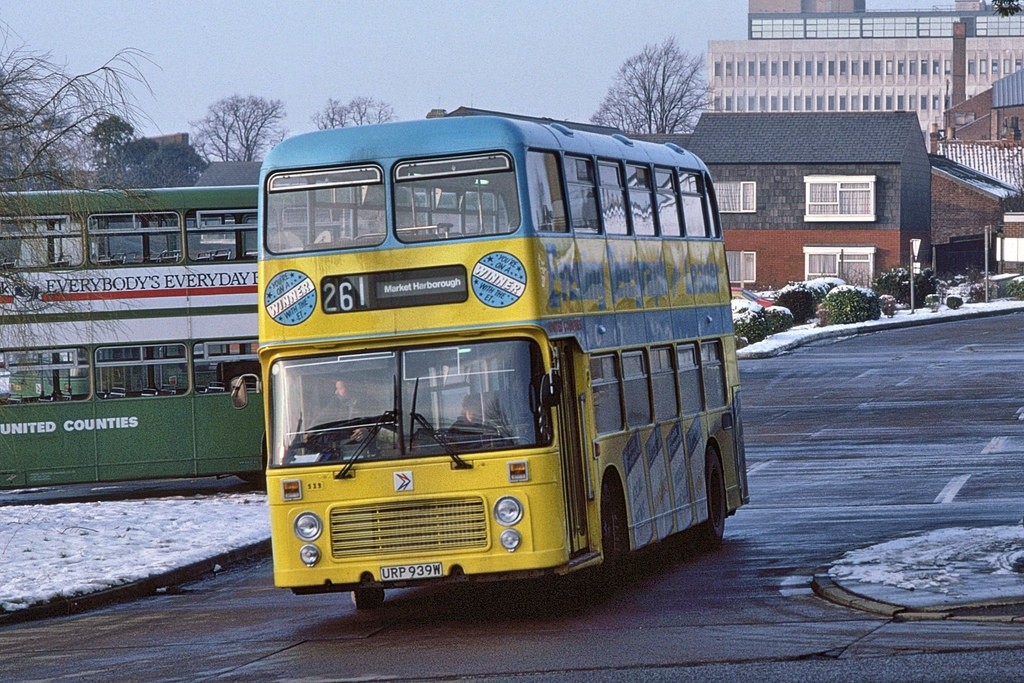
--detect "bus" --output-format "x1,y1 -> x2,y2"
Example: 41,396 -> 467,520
230,115 -> 752,609
0,183 -> 500,493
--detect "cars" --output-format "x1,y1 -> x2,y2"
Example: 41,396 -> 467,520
729,287 -> 774,308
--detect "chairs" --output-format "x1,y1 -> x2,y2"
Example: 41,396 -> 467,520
7,380 -> 257,406
0,248 -> 261,268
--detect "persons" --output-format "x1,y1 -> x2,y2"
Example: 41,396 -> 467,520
303,375 -> 380,442
446,393 -> 505,448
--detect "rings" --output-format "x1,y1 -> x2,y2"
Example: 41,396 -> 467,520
358,436 -> 360,439
